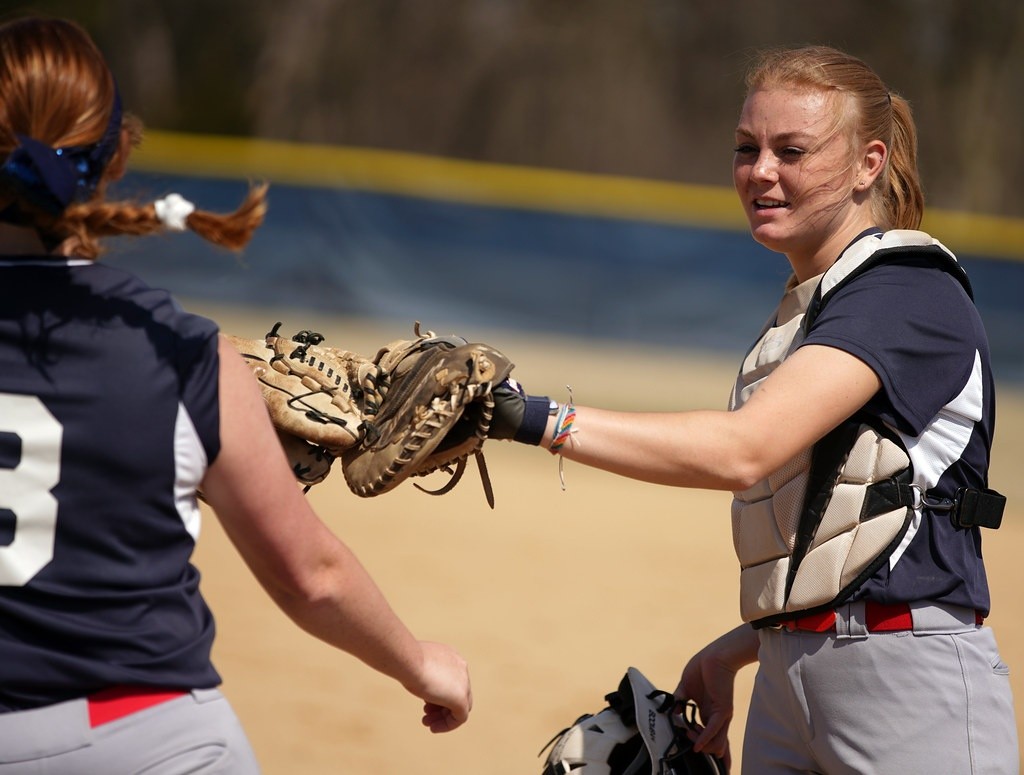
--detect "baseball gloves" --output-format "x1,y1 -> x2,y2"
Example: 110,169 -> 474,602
338,333 -> 553,503
193,333 -> 396,515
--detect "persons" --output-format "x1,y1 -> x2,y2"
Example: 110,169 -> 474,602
342,48 -> 1024,774
0,21 -> 477,773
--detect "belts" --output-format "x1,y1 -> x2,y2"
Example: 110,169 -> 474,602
767,604 -> 986,633
83,679 -> 195,730
